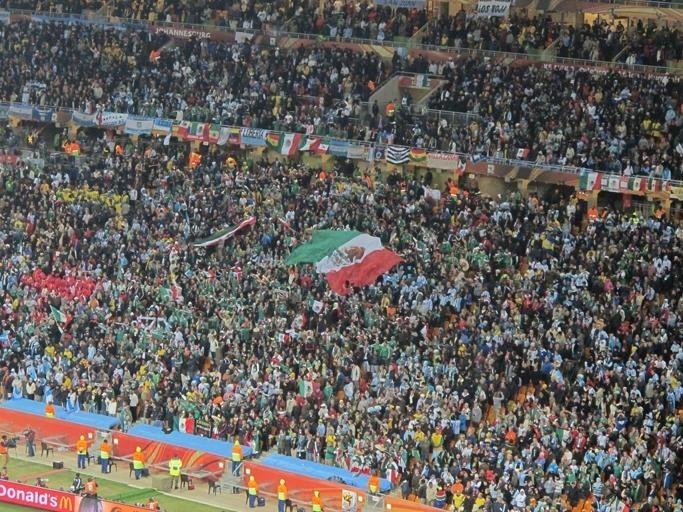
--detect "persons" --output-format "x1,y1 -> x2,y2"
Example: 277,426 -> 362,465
1,0 -> 683,512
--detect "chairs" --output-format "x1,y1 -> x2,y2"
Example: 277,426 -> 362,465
129,462 -> 139,478
41,441 -> 54,457
26,440 -> 36,453
85,451 -> 95,465
245,489 -> 258,504
181,474 -> 192,489
208,478 -> 221,496
6,439 -> 17,456
285,499 -> 298,512
108,458 -> 117,472
